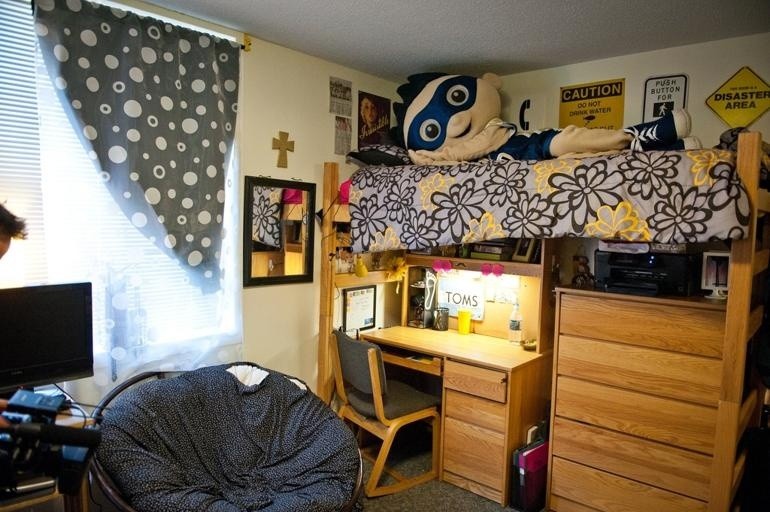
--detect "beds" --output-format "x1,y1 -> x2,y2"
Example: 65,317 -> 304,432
317,122 -> 770,510
250,185 -> 307,281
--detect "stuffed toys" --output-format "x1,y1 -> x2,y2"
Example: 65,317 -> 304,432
387,67 -> 701,163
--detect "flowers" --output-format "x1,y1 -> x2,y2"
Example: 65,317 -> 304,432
383,255 -> 409,283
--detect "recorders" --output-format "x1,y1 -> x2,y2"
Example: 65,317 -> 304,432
594,248 -> 702,298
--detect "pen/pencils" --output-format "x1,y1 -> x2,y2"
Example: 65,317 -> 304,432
433,311 -> 444,330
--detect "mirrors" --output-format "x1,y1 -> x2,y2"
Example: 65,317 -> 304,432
242,172 -> 315,290
701,251 -> 730,302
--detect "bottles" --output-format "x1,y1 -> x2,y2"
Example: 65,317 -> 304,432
509,303 -> 521,346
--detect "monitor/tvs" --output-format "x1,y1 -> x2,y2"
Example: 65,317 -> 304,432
0,282 -> 94,400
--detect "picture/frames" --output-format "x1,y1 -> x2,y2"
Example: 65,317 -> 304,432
510,236 -> 537,262
342,284 -> 379,335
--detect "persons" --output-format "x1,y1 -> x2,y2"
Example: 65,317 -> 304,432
0,204 -> 30,431
334,116 -> 353,132
330,80 -> 351,100
360,94 -> 382,136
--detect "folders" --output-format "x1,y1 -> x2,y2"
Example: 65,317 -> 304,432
512,437 -> 549,507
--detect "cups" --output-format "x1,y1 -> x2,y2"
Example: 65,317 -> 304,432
457,308 -> 472,336
432,308 -> 449,331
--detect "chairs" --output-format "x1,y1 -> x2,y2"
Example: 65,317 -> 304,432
329,332 -> 441,500
88,362 -> 364,512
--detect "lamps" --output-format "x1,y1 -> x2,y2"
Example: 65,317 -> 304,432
354,252 -> 369,280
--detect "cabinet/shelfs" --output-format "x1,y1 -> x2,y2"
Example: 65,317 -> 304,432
546,284 -> 742,509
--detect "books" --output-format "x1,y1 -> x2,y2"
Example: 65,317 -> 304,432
406,238 -> 516,261
7,387 -> 65,419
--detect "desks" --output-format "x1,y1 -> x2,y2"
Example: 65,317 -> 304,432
1,387 -> 93,512
352,236 -> 562,510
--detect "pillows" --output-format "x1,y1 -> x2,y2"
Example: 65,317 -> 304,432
345,144 -> 415,171
